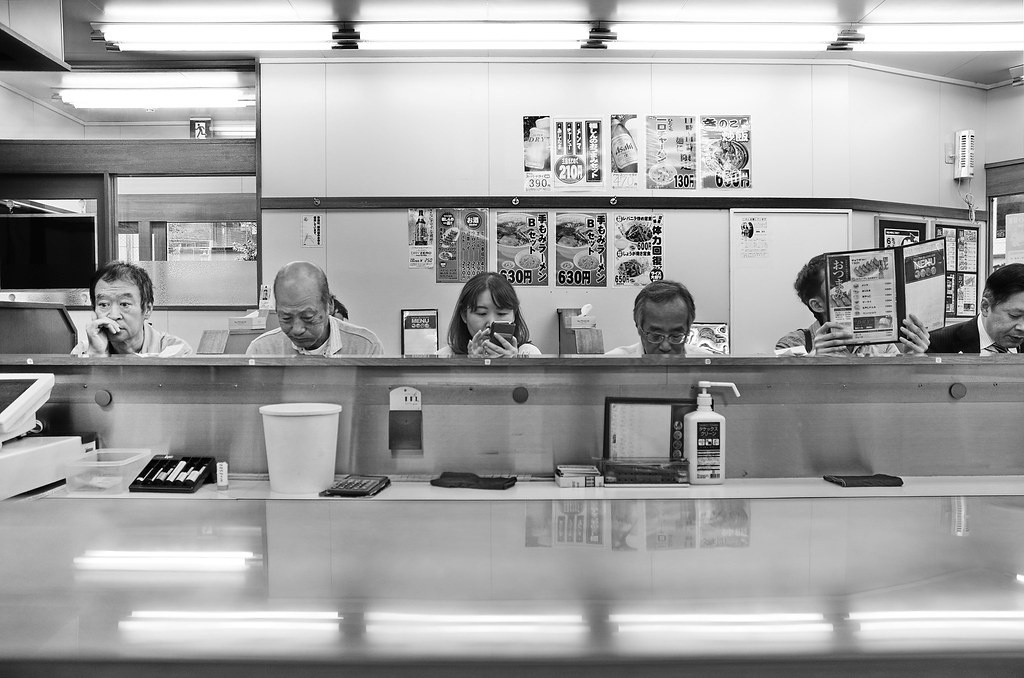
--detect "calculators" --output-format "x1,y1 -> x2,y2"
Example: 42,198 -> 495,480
318,474 -> 391,497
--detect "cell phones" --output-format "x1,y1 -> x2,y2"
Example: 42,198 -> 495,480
489,321 -> 516,350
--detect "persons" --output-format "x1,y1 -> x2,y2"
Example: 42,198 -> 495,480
775,252 -> 930,356
69,260 -> 195,357
433,272 -> 542,357
927,263 -> 1024,353
246,261 -> 385,356
602,281 -> 713,357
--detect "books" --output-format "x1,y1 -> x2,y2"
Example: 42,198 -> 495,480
823,236 -> 946,346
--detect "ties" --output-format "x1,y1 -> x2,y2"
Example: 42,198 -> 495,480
992,342 -> 1008,353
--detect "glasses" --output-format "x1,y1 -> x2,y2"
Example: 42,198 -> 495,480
640,327 -> 689,344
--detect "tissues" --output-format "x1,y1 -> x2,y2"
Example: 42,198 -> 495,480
571,304 -> 596,329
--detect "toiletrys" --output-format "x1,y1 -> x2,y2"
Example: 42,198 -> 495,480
683,381 -> 741,484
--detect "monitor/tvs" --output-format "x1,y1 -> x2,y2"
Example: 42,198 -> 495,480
0,374 -> 54,434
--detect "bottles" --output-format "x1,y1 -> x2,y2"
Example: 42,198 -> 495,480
414,210 -> 428,246
611,115 -> 640,173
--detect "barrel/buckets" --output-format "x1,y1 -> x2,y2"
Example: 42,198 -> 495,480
258,402 -> 342,493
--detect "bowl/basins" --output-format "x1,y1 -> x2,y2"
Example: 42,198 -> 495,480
706,140 -> 749,176
556,214 -> 600,258
573,251 -> 604,271
560,261 -> 574,271
502,261 -> 514,269
497,213 -> 547,258
646,162 -> 679,187
510,248 -> 545,268
